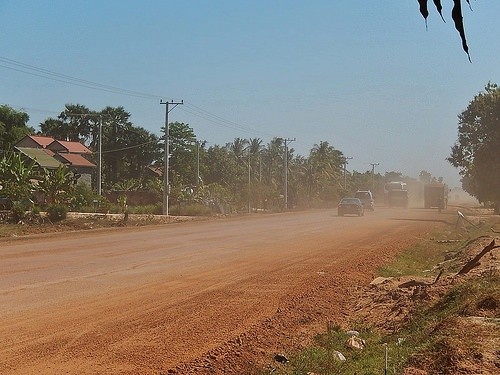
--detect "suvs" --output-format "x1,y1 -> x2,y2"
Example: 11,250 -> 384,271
354,190 -> 374,209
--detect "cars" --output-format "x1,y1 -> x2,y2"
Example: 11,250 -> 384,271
338,198 -> 364,217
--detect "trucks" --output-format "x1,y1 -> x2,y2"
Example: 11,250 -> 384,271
423,181 -> 450,209
387,180 -> 410,209
